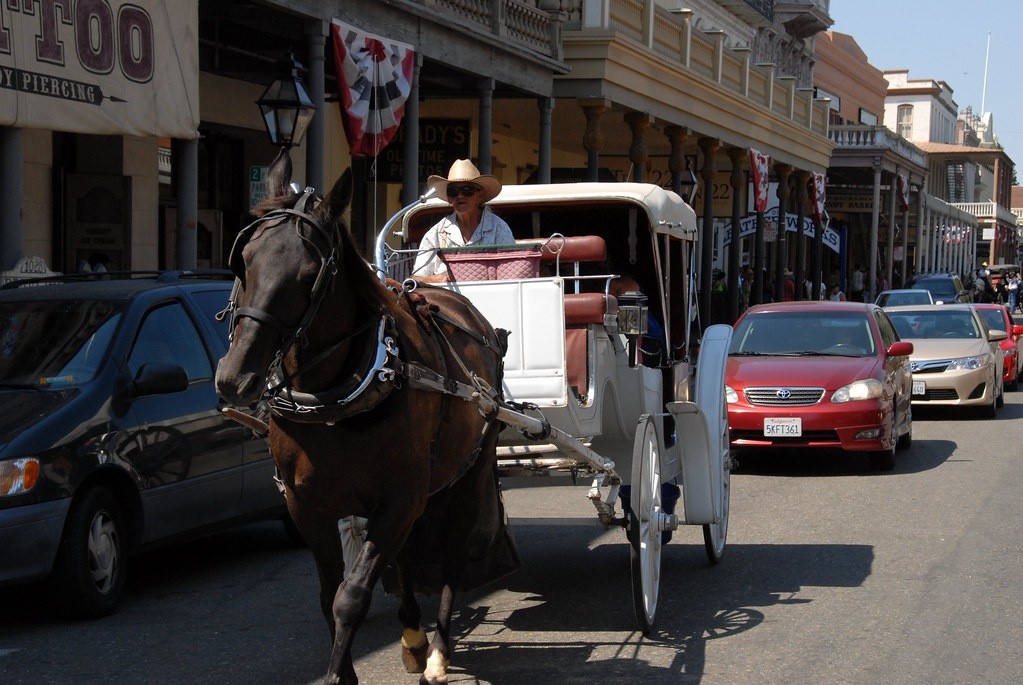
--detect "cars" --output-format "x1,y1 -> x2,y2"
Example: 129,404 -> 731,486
875,271 -> 1023,416
0,270 -> 304,608
725,300 -> 914,471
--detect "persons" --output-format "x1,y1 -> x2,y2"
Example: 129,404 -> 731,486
1002,273 -> 1023,315
713,267 -> 727,324
737,260 -> 774,318
413,158 -> 515,283
849,264 -> 915,303
784,268 -> 847,302
965,261 -> 991,303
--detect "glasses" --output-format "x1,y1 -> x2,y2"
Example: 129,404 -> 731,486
447,185 -> 480,198
749,274 -> 754,276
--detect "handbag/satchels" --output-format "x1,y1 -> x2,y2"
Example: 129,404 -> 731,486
1008,283 -> 1018,290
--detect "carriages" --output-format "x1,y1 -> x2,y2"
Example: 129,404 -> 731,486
214,182 -> 732,685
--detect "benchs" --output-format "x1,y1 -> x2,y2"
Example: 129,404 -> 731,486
516,235 -> 608,395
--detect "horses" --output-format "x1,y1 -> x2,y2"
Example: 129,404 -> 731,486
212,145 -> 520,684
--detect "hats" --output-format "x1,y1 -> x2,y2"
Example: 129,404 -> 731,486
982,262 -> 988,267
783,267 -> 794,276
428,158 -> 502,203
748,269 -> 754,274
